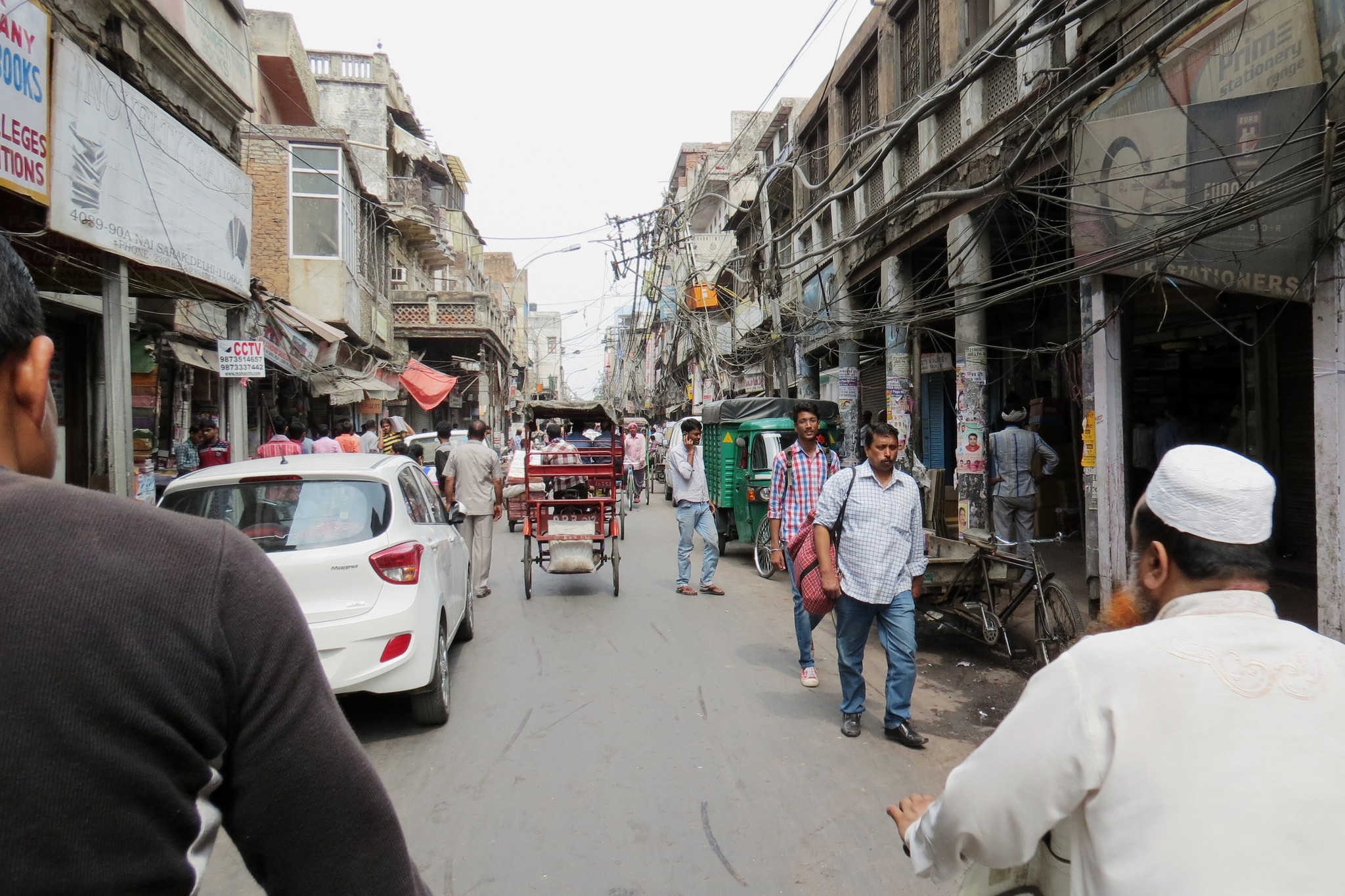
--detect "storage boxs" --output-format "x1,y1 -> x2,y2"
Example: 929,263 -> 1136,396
686,281 -> 719,311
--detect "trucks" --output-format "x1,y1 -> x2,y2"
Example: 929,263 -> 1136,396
699,396 -> 848,550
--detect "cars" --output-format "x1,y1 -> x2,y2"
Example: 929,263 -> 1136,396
400,430 -> 489,509
146,451 -> 479,730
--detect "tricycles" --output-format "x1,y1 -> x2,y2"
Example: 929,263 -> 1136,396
612,416 -> 652,510
516,397 -> 626,600
904,526 -> 1079,679
503,448 -> 552,530
655,438 -> 669,484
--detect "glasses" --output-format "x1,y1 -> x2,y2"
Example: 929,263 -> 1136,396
796,419 -> 819,423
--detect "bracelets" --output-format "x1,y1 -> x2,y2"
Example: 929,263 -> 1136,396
688,454 -> 694,455
770,549 -> 783,552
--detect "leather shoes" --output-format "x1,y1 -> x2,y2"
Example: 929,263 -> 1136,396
885,723 -> 929,747
840,712 -> 861,737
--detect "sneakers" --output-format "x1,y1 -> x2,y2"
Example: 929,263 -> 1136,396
801,666 -> 819,687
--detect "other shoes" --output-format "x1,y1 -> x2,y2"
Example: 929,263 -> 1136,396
619,484 -> 622,489
634,499 -> 640,503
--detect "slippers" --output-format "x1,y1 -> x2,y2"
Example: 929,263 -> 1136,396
477,589 -> 491,597
700,586 -> 725,595
676,588 -> 698,595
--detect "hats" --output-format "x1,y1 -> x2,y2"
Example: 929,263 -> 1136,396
628,422 -> 638,429
1144,444 -> 1277,545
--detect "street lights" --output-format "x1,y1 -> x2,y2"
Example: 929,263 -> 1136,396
506,242 -> 581,442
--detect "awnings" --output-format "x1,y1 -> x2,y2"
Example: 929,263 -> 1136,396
307,367 -> 399,405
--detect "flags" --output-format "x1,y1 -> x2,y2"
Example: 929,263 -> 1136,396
399,357 -> 457,411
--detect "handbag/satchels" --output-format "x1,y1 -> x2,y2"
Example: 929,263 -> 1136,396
786,507 -> 841,615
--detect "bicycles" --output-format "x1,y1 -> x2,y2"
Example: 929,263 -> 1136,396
753,510 -> 786,577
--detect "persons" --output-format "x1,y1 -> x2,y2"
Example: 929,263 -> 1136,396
891,396 -> 913,451
886,440 -> 1345,895
858,410 -> 872,462
765,401 -> 841,688
957,373 -> 983,528
670,418 -> 725,596
174,408 -> 672,551
813,422 -> 928,747
843,401 -> 850,408
986,402 -> 1060,586
877,410 -> 887,423
0,229 -> 431,895
